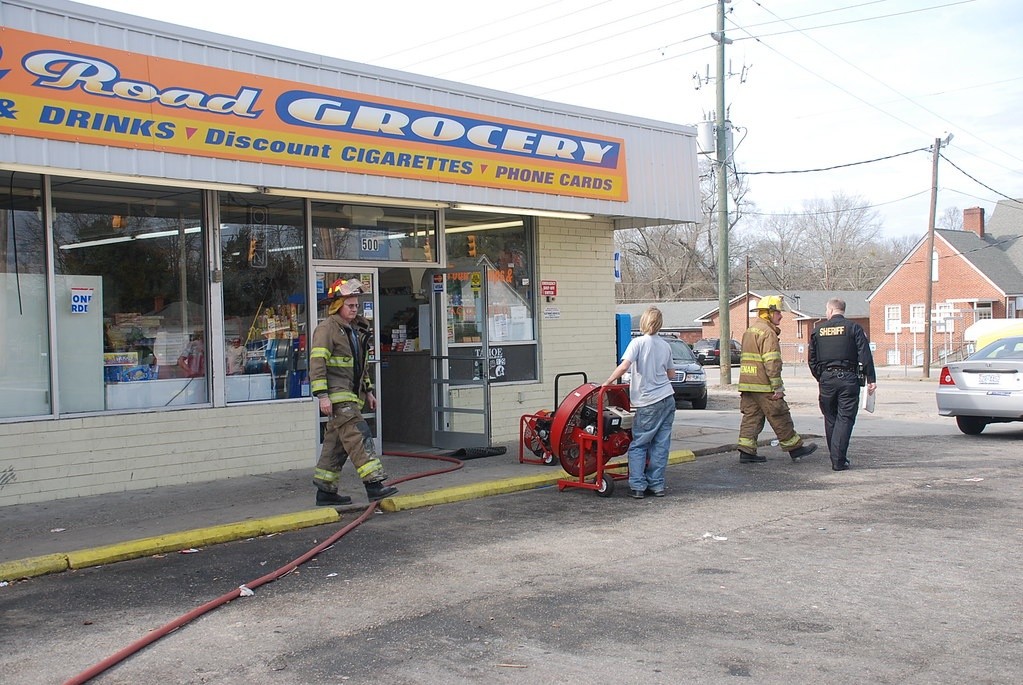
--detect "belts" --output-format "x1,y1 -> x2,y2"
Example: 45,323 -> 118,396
825,367 -> 855,372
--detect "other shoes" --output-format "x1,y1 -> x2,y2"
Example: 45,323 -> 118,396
627,486 -> 644,498
645,489 -> 664,497
832,458 -> 850,470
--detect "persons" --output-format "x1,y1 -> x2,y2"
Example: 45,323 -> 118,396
177,331 -> 205,377
309,278 -> 399,506
124,341 -> 157,381
737,296 -> 817,463
227,337 -> 247,376
601,305 -> 676,499
808,298 -> 877,471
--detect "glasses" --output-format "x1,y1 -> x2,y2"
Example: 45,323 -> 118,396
234,341 -> 240,344
344,304 -> 360,309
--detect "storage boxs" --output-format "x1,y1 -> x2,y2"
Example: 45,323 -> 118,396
391,325 -> 415,352
104,352 -> 159,382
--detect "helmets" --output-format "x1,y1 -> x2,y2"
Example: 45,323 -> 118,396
319,276 -> 372,315
750,294 -> 784,313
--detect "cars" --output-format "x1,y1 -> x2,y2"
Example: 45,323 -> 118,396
935,336 -> 1023,435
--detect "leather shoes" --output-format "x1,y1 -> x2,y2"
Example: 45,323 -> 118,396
740,451 -> 766,463
789,441 -> 818,461
316,488 -> 352,506
365,480 -> 399,502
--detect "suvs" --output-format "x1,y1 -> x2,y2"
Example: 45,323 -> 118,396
691,338 -> 742,366
632,331 -> 708,410
